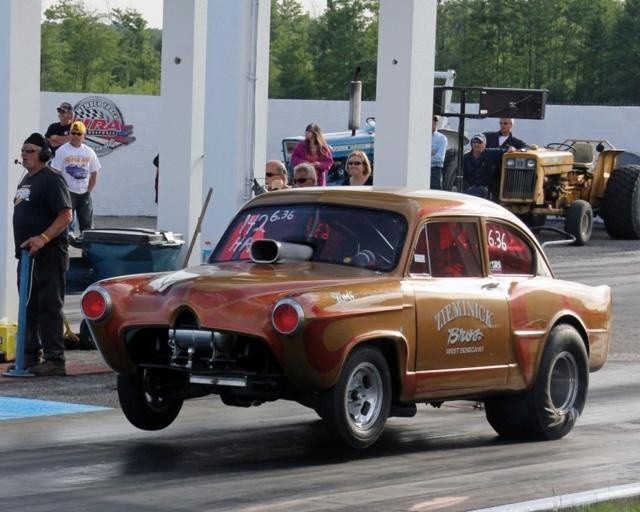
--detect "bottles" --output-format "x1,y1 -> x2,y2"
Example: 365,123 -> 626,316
202,240 -> 212,263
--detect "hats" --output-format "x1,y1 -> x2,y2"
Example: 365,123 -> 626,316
23,132 -> 47,147
472,132 -> 488,145
69,120 -> 87,135
57,102 -> 73,113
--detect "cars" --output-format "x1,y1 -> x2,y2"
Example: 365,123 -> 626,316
80,180 -> 615,455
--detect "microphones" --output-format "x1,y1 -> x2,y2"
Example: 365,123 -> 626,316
15,159 -> 21,164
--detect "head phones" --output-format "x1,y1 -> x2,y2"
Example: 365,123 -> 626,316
40,135 -> 52,162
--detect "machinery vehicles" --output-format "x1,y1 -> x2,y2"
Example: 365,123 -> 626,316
463,131 -> 640,248
278,68 -> 471,197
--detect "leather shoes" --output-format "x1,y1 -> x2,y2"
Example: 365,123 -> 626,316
6,358 -> 42,372
28,360 -> 67,378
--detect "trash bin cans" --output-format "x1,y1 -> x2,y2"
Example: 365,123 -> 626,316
63,226 -> 187,296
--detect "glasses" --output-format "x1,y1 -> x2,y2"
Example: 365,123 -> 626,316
347,160 -> 363,166
293,176 -> 312,185
21,149 -> 37,155
500,121 -> 509,126
265,171 -> 281,178
70,132 -> 83,137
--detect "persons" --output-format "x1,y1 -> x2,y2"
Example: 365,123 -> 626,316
50,120 -> 102,233
41,233 -> 50,243
45,102 -> 77,230
431,115 -> 447,190
462,133 -> 499,199
7,133 -> 73,378
486,117 -> 530,155
251,122 -> 374,196
